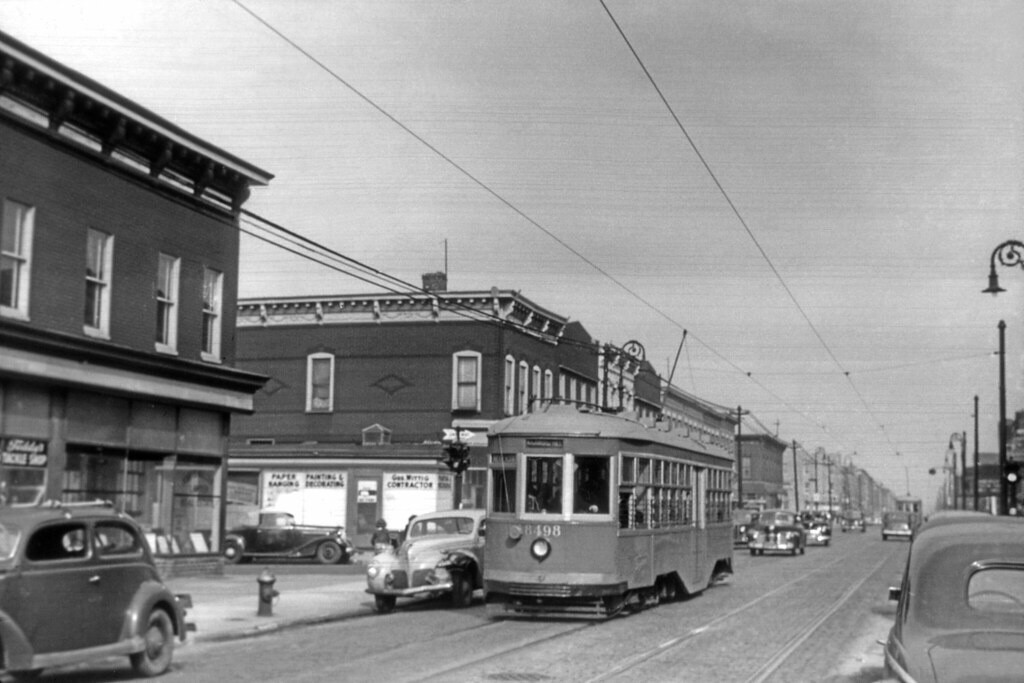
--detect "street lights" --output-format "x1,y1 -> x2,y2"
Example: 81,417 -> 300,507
949,432 -> 967,508
980,240 -> 1024,298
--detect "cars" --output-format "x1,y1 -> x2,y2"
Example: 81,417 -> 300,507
803,513 -> 831,547
747,510 -> 807,554
881,513 -> 914,539
0,500 -> 195,683
223,510 -> 355,563
840,511 -> 865,532
365,508 -> 483,612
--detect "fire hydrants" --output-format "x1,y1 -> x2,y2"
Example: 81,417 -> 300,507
257,568 -> 279,616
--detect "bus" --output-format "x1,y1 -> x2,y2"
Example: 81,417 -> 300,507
481,408 -> 735,606
